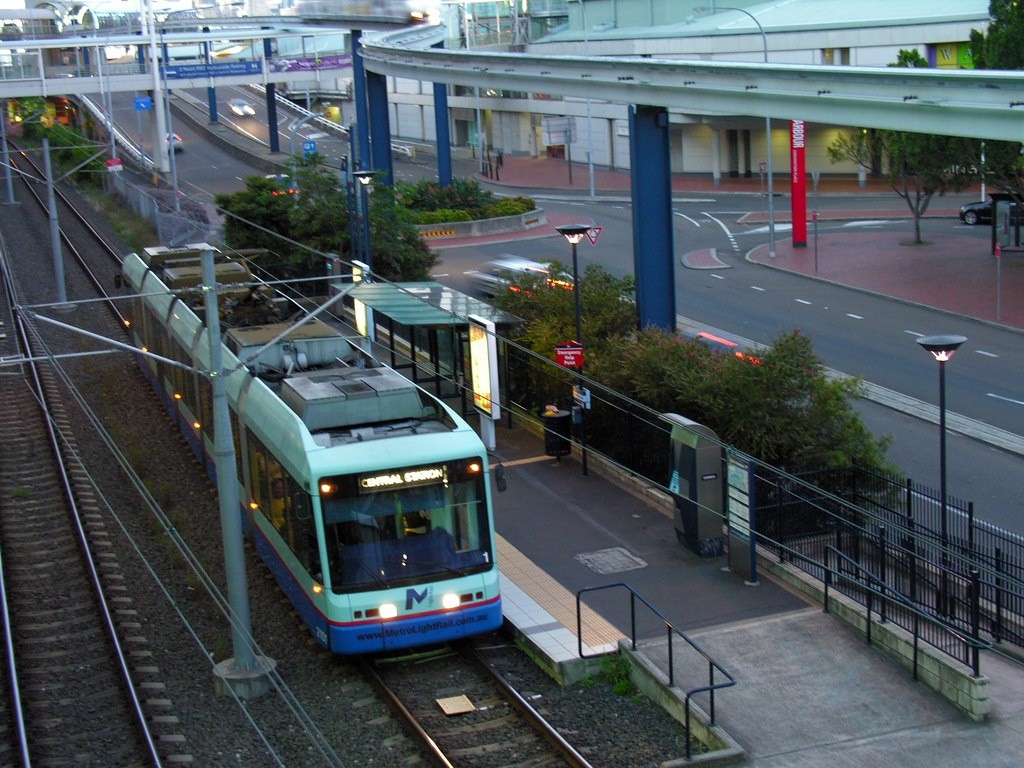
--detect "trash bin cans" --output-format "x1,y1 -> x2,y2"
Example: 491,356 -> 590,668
542,410 -> 572,457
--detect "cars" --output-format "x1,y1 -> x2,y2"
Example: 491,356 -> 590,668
165,133 -> 186,154
958,192 -> 1024,224
229,98 -> 256,119
264,172 -> 298,198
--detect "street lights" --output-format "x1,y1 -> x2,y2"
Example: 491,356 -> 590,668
292,112 -> 328,212
551,224 -> 594,477
686,5 -> 776,258
915,334 -> 970,618
160,1 -> 247,212
352,170 -> 377,342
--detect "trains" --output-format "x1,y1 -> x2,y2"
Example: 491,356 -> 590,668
114,243 -> 509,658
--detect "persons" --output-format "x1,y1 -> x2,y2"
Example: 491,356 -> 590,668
258,447 -> 292,533
404,509 -> 431,536
